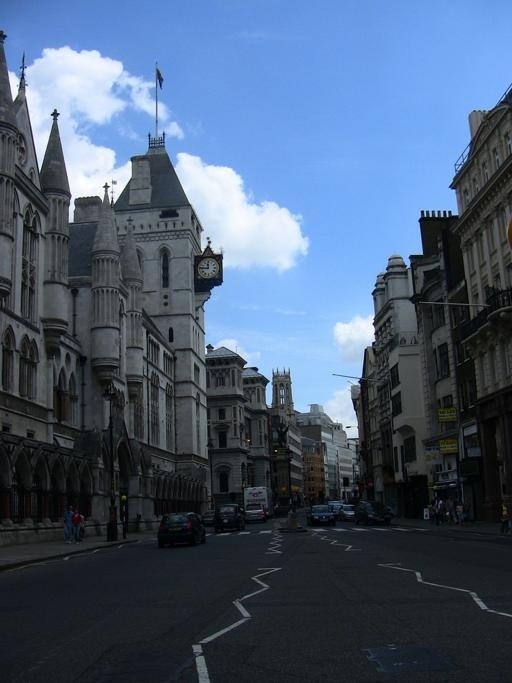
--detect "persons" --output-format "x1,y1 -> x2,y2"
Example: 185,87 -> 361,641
62,504 -> 86,545
499,500 -> 510,533
428,494 -> 465,528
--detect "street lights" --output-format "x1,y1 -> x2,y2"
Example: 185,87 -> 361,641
285,445 -> 297,530
102,378 -> 117,541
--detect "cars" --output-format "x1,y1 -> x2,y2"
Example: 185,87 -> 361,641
273,496 -> 290,516
200,509 -> 214,523
213,504 -> 246,532
157,512 -> 204,547
328,500 -> 394,525
306,505 -> 337,527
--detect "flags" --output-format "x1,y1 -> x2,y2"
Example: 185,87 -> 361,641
157,68 -> 164,89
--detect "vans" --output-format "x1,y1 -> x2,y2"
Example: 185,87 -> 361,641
242,502 -> 266,522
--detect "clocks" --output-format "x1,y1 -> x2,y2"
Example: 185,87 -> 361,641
194,236 -> 222,283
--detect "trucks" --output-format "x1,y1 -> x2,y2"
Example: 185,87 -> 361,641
243,485 -> 272,515
